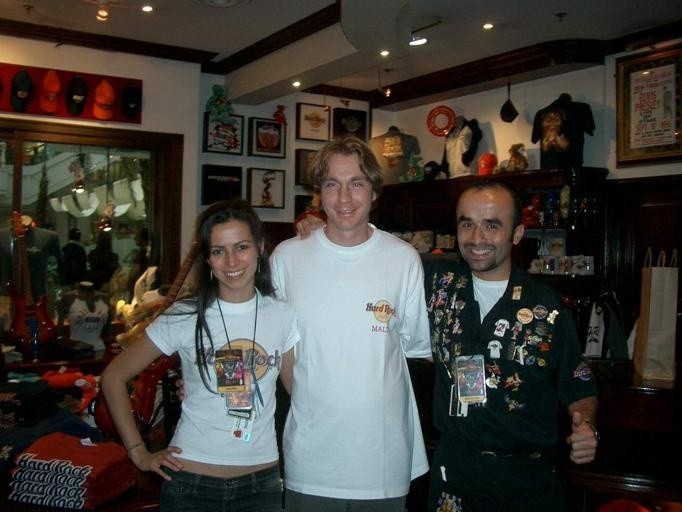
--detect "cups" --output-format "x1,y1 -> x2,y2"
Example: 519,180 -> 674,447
529,255 -> 597,275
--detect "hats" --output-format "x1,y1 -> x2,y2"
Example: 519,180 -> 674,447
9,70 -> 32,111
38,70 -> 60,113
93,79 -> 113,119
424,161 -> 441,180
65,76 -> 88,116
121,83 -> 140,118
478,153 -> 497,176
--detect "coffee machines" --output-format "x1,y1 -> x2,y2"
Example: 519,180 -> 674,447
512,237 -> 540,272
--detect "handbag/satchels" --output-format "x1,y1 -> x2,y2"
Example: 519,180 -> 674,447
633,248 -> 679,381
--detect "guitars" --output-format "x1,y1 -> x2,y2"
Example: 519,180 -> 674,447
93,241 -> 200,432
9,211 -> 56,352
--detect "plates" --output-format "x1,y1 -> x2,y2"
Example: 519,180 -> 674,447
427,106 -> 454,137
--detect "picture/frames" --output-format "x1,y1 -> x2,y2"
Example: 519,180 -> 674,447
296,102 -> 331,144
248,117 -> 286,160
202,111 -> 244,156
246,167 -> 285,209
295,148 -> 317,186
615,42 -> 682,169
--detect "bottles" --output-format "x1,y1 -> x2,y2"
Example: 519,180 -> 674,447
574,295 -> 593,353
546,197 -> 598,229
29,333 -> 40,359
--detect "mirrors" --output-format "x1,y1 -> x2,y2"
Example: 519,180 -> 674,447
0,129 -> 164,338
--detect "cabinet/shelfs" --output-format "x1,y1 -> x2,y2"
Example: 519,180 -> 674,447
515,181 -> 595,284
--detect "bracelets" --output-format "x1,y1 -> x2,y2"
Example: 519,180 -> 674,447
585,420 -> 600,441
127,442 -> 145,452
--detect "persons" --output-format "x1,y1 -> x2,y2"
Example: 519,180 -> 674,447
267,137 -> 433,511
509,144 -> 529,171
57,281 -> 113,357
296,178 -> 602,512
98,198 -> 300,511
531,92 -> 596,168
369,126 -> 420,183
662,85 -> 675,119
1,216 -> 62,311
59,223 -> 161,300
441,115 -> 482,178
